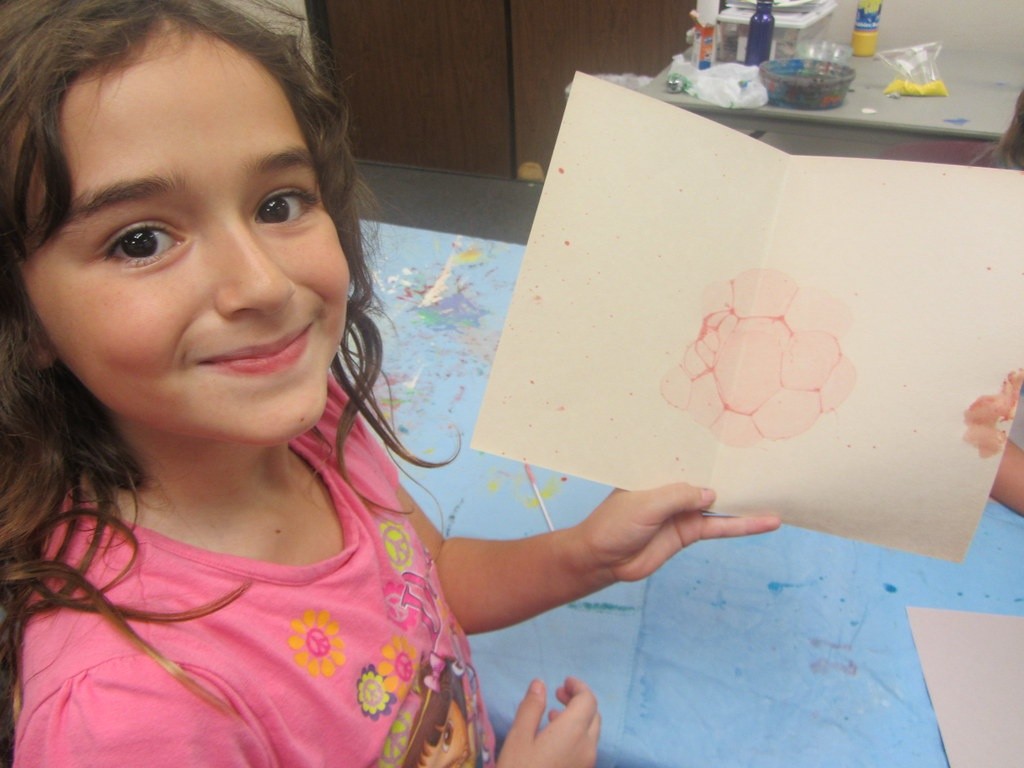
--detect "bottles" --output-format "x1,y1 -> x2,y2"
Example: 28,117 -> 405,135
852,0 -> 883,57
745,0 -> 774,65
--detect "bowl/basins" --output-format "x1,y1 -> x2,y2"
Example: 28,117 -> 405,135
760,59 -> 855,109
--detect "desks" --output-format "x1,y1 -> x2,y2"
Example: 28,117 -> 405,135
627,49 -> 1024,168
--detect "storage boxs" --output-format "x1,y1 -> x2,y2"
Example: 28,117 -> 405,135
716,0 -> 838,59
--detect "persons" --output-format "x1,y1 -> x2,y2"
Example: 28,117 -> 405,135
0,0 -> 780,768
